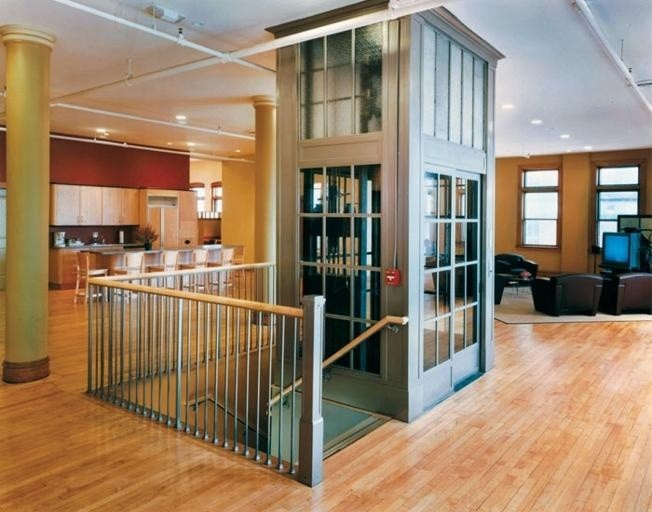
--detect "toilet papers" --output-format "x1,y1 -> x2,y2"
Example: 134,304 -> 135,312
119,231 -> 124,243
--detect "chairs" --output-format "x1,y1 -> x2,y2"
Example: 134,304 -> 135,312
494,253 -> 652,316
73,244 -> 246,305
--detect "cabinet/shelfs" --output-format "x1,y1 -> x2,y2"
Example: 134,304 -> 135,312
49,182 -> 198,289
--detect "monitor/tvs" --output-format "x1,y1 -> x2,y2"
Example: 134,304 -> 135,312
601,232 -> 651,270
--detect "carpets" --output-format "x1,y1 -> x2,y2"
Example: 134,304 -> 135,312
494,287 -> 652,324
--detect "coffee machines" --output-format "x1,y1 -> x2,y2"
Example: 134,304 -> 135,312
53,230 -> 66,248
